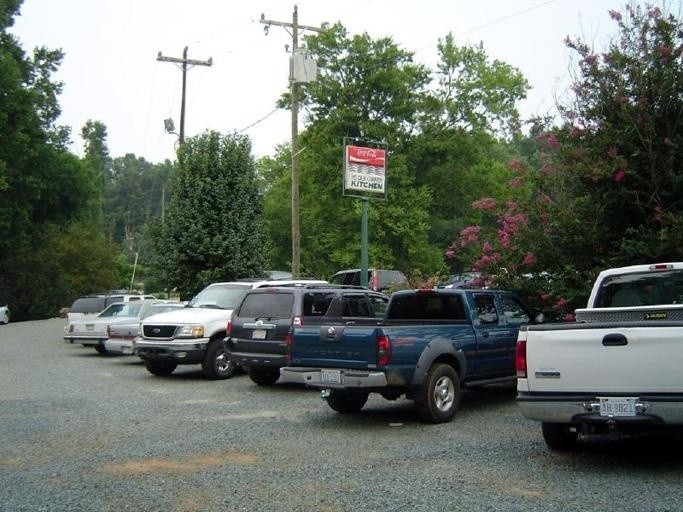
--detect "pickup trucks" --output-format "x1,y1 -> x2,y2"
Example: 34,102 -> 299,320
275,288 -> 536,424
514,263 -> 682,451
